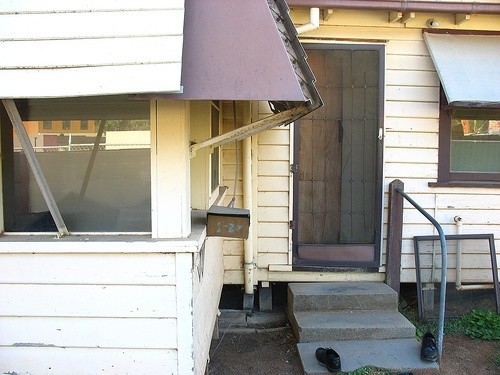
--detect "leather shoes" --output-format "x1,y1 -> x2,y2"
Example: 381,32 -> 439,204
316,348 -> 341,372
420,333 -> 439,363
